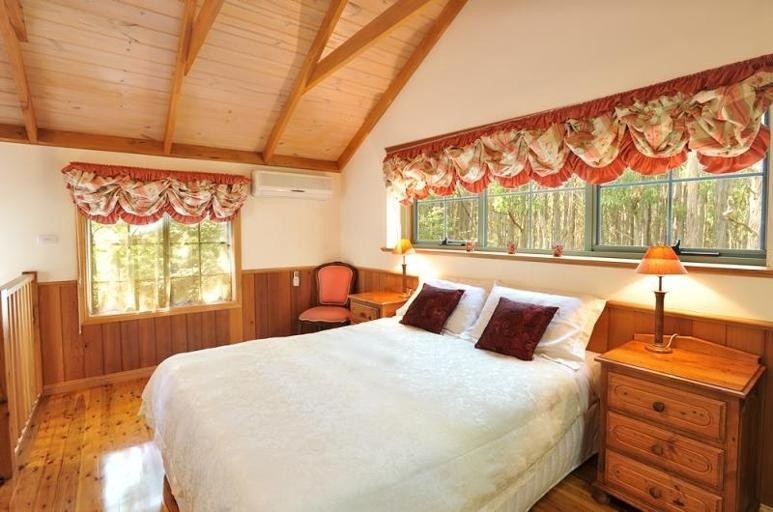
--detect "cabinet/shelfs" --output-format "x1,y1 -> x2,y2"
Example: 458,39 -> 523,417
347,288 -> 410,324
594,334 -> 766,511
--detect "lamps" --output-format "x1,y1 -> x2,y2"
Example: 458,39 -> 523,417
391,239 -> 417,297
634,246 -> 688,353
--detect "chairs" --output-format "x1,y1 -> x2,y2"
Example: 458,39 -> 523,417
297,261 -> 357,334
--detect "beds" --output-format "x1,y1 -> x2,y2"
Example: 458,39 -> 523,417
141,276 -> 602,511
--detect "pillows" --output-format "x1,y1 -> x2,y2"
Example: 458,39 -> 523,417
397,276 -> 607,369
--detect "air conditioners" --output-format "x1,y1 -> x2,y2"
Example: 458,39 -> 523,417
250,169 -> 332,202
